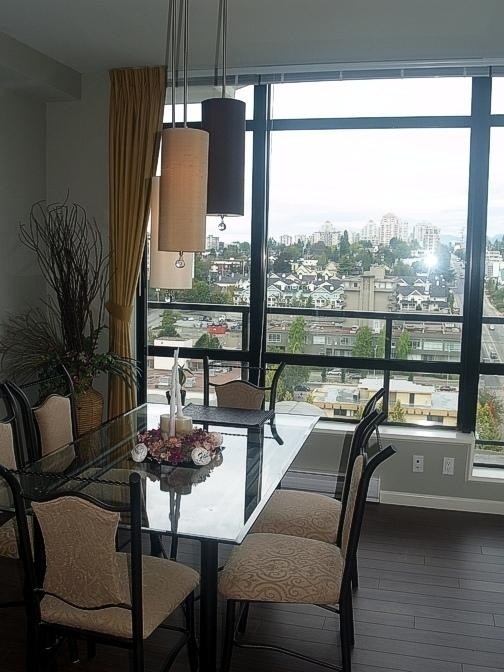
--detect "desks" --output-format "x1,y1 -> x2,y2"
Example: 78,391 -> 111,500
0,403 -> 322,672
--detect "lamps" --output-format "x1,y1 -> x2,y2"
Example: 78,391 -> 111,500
157,1 -> 210,269
147,1 -> 196,291
200,0 -> 245,232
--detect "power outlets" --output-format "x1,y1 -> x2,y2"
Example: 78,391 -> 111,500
441,457 -> 456,475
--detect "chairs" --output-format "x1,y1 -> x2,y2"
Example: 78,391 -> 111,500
217,409 -> 397,670
0,381 -> 54,649
201,352 -> 289,409
2,362 -> 168,559
247,386 -> 386,590
201,410 -> 283,445
0,465 -> 202,672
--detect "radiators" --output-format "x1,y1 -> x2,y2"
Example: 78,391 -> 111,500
280,469 -> 380,503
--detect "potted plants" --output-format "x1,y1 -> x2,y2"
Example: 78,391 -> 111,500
0,184 -> 151,471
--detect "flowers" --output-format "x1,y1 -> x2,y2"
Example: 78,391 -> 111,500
128,451 -> 223,487
130,423 -> 223,465
